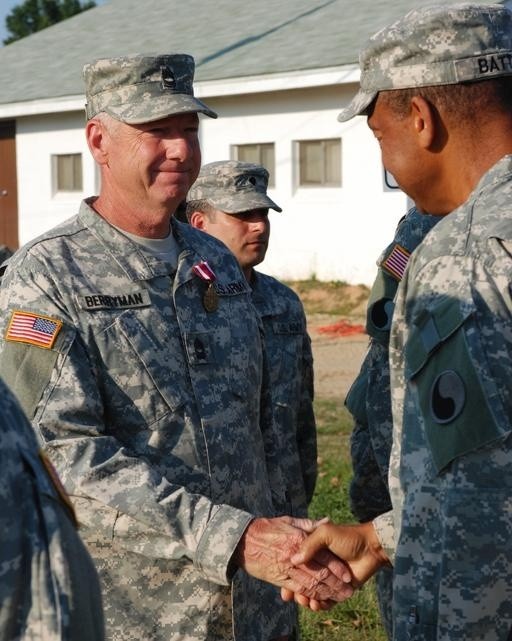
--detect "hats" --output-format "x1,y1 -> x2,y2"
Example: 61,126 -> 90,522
82,55 -> 217,125
337,3 -> 511,122
185,160 -> 281,215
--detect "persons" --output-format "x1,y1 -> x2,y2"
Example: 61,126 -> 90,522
279,4 -> 510,640
0,377 -> 103,639
1,52 -> 354,640
180,161 -> 316,507
344,208 -> 441,630
